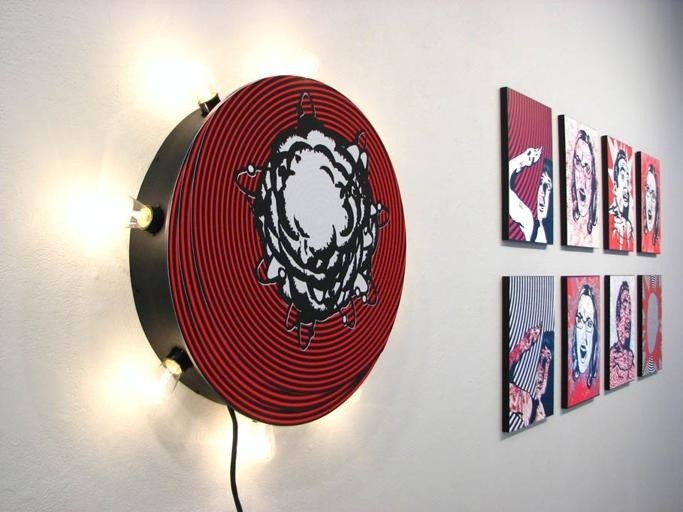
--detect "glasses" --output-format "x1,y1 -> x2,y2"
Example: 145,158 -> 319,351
575,313 -> 595,333
573,155 -> 593,179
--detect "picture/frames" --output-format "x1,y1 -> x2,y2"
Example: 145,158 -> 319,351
639,271 -> 662,380
502,272 -> 557,432
603,134 -> 636,255
559,272 -> 603,410
606,271 -> 639,394
558,113 -> 604,251
636,149 -> 662,256
503,87 -> 556,251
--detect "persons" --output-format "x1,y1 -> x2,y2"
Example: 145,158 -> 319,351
570,283 -> 602,407
508,322 -> 553,431
611,279 -> 634,389
568,129 -> 599,247
644,164 -> 662,254
608,150 -> 637,247
509,143 -> 553,244
